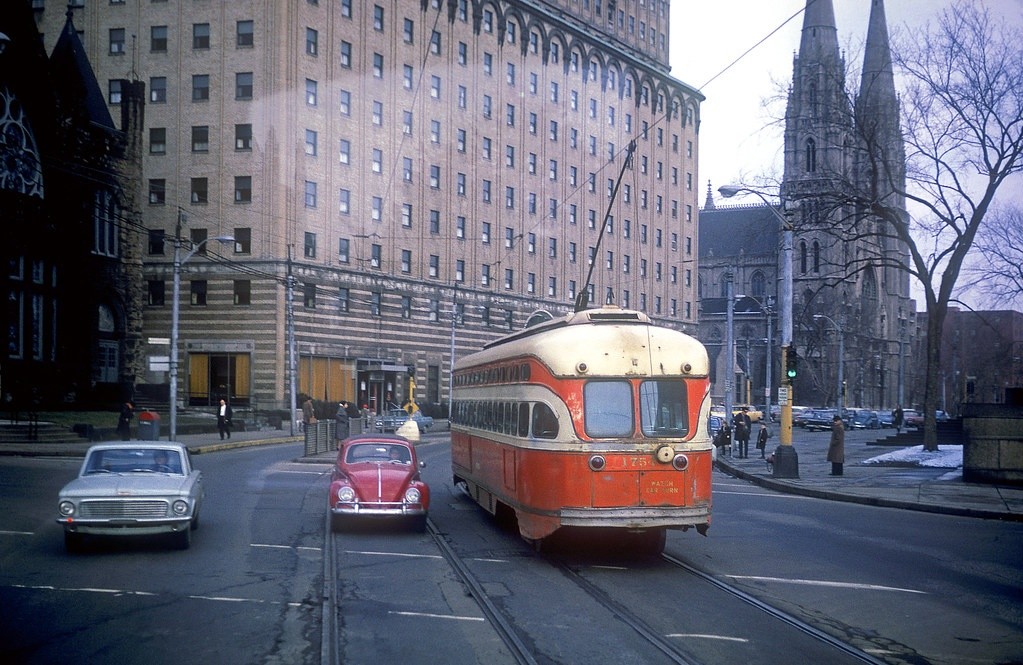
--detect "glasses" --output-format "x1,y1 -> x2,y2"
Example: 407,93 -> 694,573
744,411 -> 748,413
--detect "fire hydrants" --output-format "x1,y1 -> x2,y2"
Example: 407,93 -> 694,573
765,452 -> 774,472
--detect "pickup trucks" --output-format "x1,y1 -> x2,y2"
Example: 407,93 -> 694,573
375,409 -> 435,433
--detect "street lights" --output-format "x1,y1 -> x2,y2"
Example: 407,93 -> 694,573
734,294 -> 772,428
941,370 -> 960,410
812,314 -> 843,420
718,185 -> 799,478
169,207 -> 236,442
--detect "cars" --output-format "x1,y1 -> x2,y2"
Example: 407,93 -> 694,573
328,433 -> 430,532
55,441 -> 204,552
711,402 -> 764,442
758,405 -> 950,431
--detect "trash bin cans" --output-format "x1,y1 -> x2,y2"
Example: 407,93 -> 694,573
136,409 -> 161,440
270,416 -> 282,430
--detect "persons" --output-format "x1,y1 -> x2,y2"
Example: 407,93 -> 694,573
361,405 -> 369,428
335,400 -> 348,451
217,400 -> 233,441
302,397 -> 315,421
721,422 -> 731,455
153,450 -> 169,465
116,401 -> 135,441
756,424 -> 768,460
826,416 -> 844,476
734,406 -> 751,459
894,404 -> 904,432
390,447 -> 402,460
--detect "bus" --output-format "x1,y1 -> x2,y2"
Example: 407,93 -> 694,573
450,140 -> 718,571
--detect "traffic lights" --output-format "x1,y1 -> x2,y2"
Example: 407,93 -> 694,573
407,365 -> 415,377
785,347 -> 797,379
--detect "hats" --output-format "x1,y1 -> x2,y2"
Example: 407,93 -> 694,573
832,416 -> 840,420
